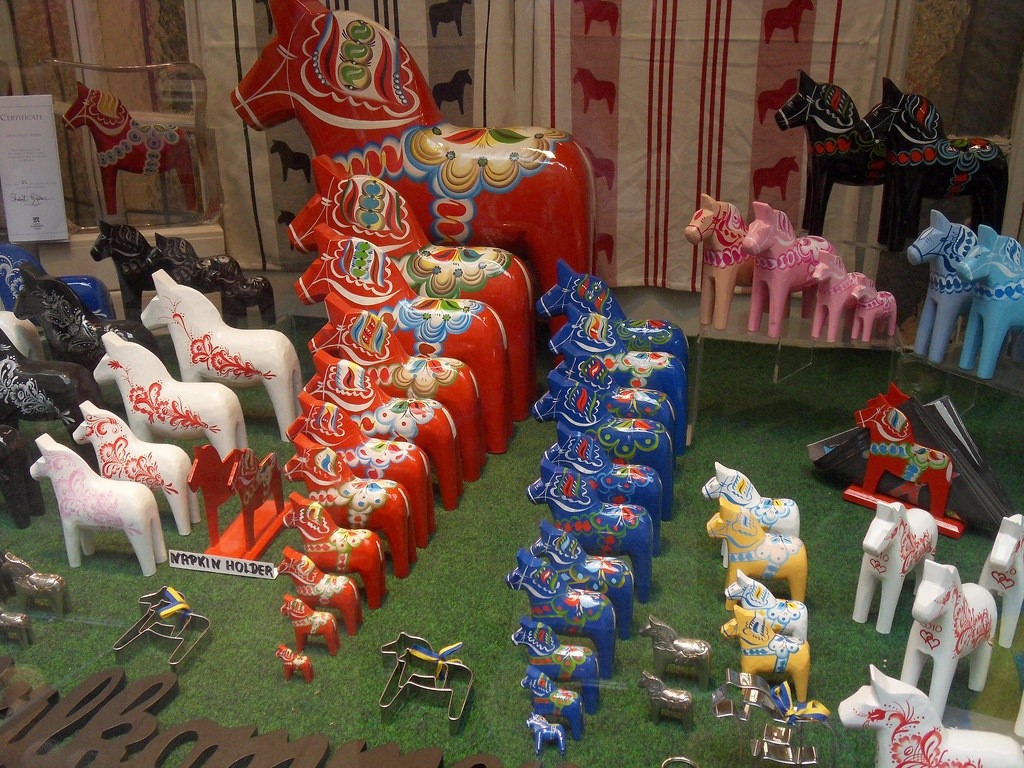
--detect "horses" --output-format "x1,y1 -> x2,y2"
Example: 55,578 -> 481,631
637,614 -> 713,733
0,552 -> 74,648
0,222 -> 305,577
837,500 -> 1024,768
230,2 -> 596,683
684,68 -> 1023,381
506,261 -> 690,756
701,462 -> 811,705
61,81 -> 198,216
